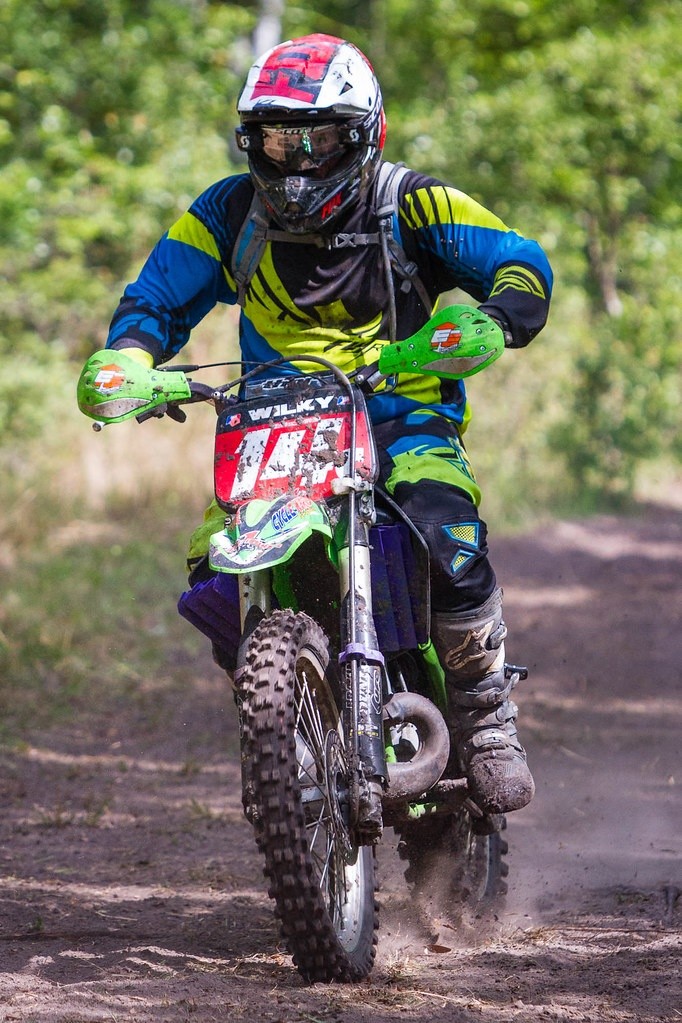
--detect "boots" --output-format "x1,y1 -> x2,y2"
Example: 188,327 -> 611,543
429,585 -> 535,813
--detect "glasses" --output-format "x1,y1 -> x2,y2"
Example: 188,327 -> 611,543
261,118 -> 345,168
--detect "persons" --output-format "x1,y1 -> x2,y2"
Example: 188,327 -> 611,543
79,36 -> 552,815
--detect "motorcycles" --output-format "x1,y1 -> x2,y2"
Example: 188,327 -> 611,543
78,303 -> 511,980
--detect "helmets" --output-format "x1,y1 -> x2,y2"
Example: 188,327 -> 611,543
236,33 -> 387,234
